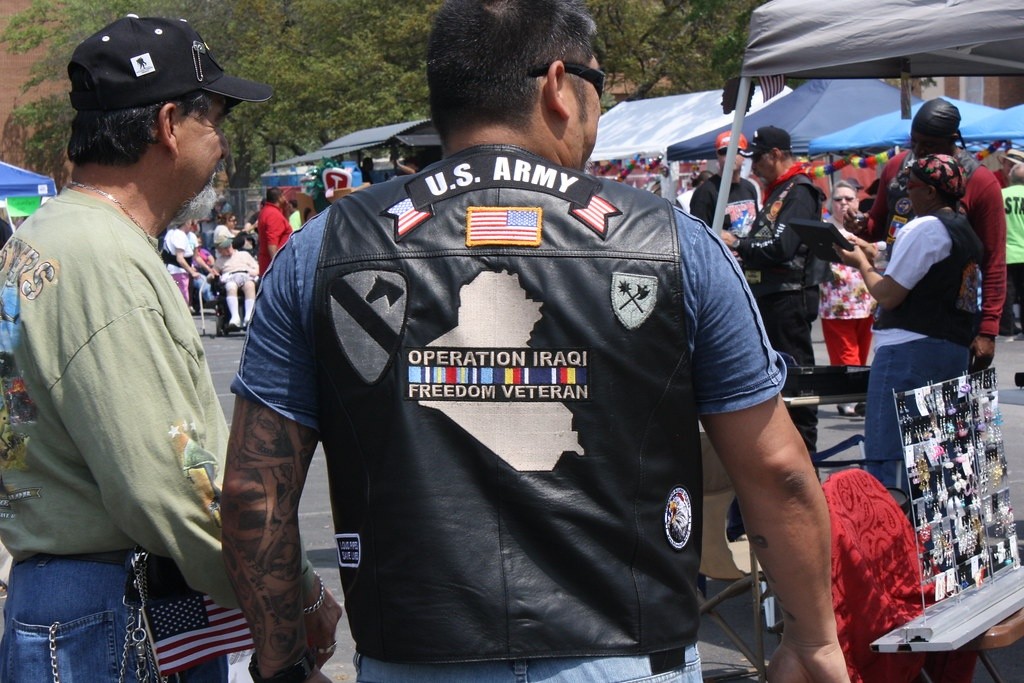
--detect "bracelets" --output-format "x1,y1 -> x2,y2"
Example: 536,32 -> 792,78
302,571 -> 326,614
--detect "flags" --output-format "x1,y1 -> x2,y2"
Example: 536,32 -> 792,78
147,594 -> 256,677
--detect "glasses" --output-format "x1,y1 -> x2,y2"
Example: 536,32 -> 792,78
716,148 -> 741,156
832,196 -> 856,202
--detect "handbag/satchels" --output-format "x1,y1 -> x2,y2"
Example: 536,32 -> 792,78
145,597 -> 255,677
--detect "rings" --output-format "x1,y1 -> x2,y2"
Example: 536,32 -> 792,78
319,641 -> 337,653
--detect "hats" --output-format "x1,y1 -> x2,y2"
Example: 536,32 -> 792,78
212,225 -> 235,249
913,98 -> 967,149
748,125 -> 791,154
68,13 -> 274,110
996,149 -> 1023,164
909,154 -> 966,197
844,178 -> 864,190
714,131 -> 748,150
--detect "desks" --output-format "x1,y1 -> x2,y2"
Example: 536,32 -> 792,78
697,389 -> 868,683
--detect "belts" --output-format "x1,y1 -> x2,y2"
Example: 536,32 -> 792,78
59,548 -> 177,570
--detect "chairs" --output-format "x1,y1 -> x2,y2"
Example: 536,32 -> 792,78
821,469 -> 1024,683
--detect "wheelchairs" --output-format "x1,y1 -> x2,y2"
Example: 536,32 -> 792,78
208,274 -> 262,337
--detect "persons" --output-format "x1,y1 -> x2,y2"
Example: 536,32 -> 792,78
166,102 -> 1024,509
224,0 -> 852,682
0,15 -> 342,683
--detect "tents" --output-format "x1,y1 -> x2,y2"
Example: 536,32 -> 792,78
1,155 -> 57,219
591,0 -> 1024,251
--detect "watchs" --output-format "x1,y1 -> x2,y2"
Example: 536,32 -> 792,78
248,649 -> 314,682
